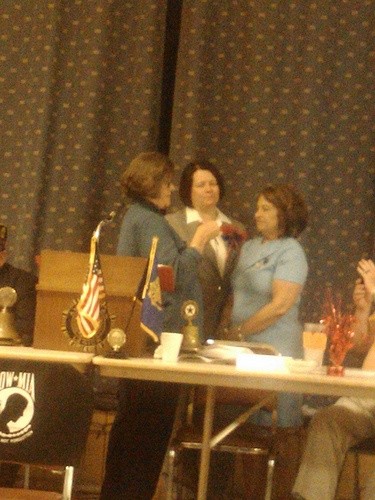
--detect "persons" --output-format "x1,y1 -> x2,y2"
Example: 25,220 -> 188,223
0,225 -> 37,489
294,259 -> 374,500
98,149 -> 308,500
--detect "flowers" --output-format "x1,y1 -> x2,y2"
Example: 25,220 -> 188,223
220,220 -> 249,253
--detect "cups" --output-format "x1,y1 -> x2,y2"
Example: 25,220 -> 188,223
303,323 -> 328,369
159,333 -> 183,364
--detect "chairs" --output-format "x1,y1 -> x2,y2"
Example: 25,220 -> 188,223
166,338 -> 281,500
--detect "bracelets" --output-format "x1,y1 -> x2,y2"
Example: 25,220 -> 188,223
237,324 -> 242,340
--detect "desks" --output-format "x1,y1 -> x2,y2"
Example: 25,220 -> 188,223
0,343 -> 375,401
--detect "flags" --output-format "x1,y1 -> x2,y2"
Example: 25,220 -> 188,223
76,223 -> 107,338
132,238 -> 169,342
